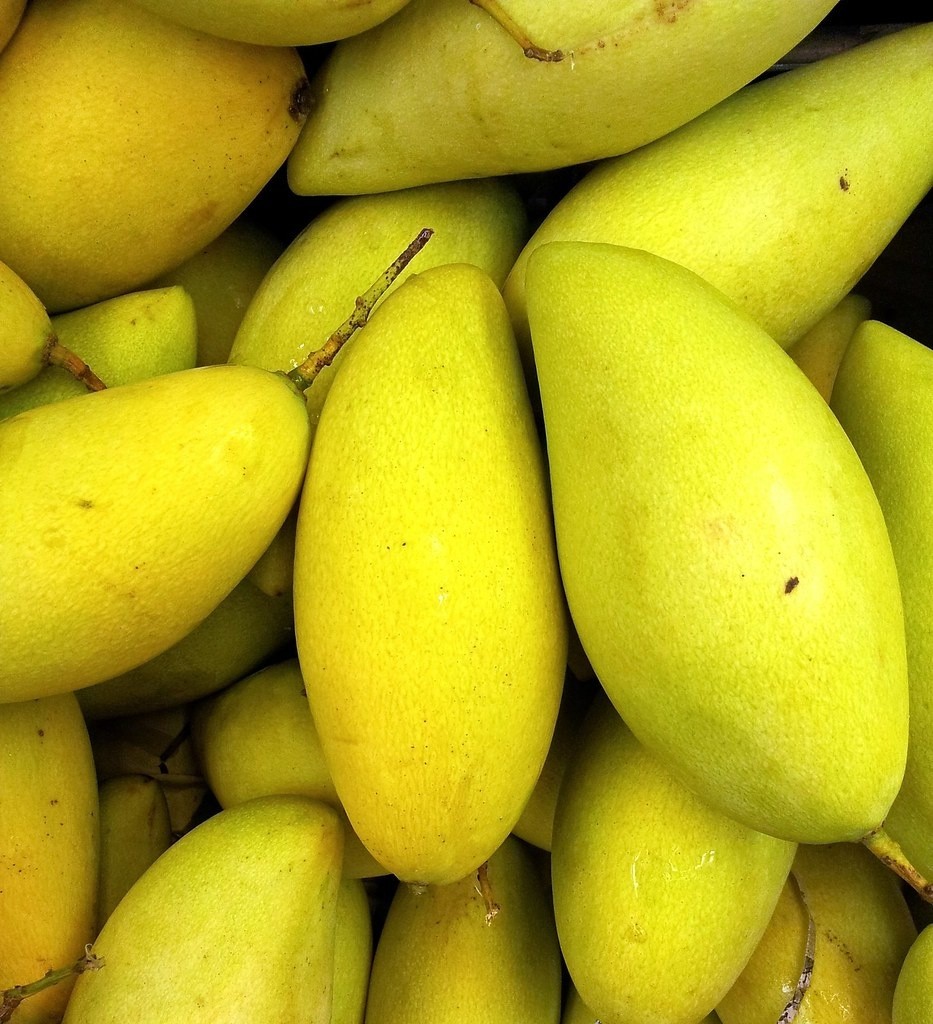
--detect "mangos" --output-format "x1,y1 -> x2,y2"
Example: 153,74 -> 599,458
0,0 -> 933,1024
